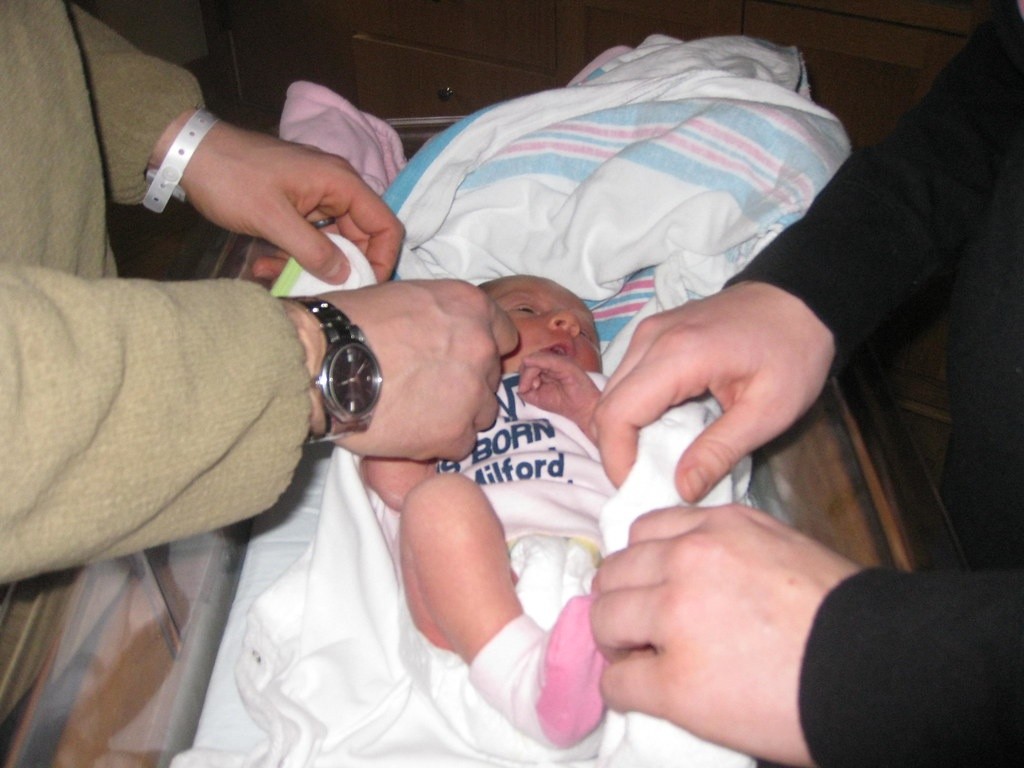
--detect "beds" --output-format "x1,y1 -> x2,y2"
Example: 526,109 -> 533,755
1,112 -> 975,768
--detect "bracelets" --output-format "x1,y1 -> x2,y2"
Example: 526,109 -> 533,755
144,106 -> 220,214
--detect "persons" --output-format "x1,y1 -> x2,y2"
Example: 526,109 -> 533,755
590,0 -> 1024,768
0,0 -> 521,768
361,274 -> 615,761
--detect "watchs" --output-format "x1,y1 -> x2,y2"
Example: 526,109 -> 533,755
275,296 -> 382,445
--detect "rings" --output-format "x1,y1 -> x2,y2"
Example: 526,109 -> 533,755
312,217 -> 333,227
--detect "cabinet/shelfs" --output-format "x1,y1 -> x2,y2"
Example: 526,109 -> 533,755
229,0 -> 997,489
